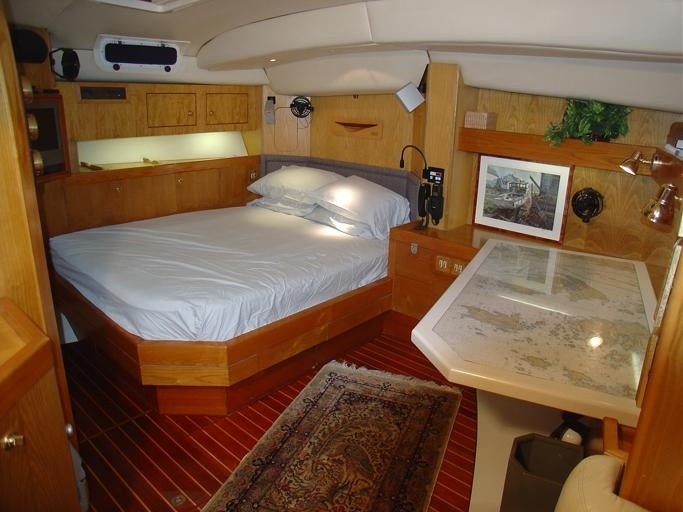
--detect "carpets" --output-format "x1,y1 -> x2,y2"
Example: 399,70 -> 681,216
200,360 -> 463,512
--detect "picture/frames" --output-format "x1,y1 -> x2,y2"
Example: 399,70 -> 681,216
472,153 -> 575,245
471,227 -> 560,296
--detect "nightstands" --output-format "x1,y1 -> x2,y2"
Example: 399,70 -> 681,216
392,240 -> 470,320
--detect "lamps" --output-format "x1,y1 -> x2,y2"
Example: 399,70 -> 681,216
640,183 -> 683,226
617,150 -> 683,176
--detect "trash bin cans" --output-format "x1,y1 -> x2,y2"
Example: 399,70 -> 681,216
499,433 -> 584,510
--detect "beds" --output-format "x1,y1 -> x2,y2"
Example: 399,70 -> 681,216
49,155 -> 421,416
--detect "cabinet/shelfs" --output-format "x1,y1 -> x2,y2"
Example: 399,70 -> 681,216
35,162 -> 259,233
206,93 -> 248,125
147,93 -> 196,128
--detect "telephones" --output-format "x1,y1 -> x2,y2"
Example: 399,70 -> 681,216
429,184 -> 444,226
415,183 -> 431,218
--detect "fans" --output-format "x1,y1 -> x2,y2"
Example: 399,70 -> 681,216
290,96 -> 314,118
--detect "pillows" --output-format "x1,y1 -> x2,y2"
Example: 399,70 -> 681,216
309,175 -> 410,240
246,197 -> 319,217
247,165 -> 346,206
303,206 -> 377,240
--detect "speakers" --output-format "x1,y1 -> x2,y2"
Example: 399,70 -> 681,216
9,27 -> 49,65
61,46 -> 80,81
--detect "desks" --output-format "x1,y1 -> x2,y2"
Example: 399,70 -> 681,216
410,238 -> 658,512
